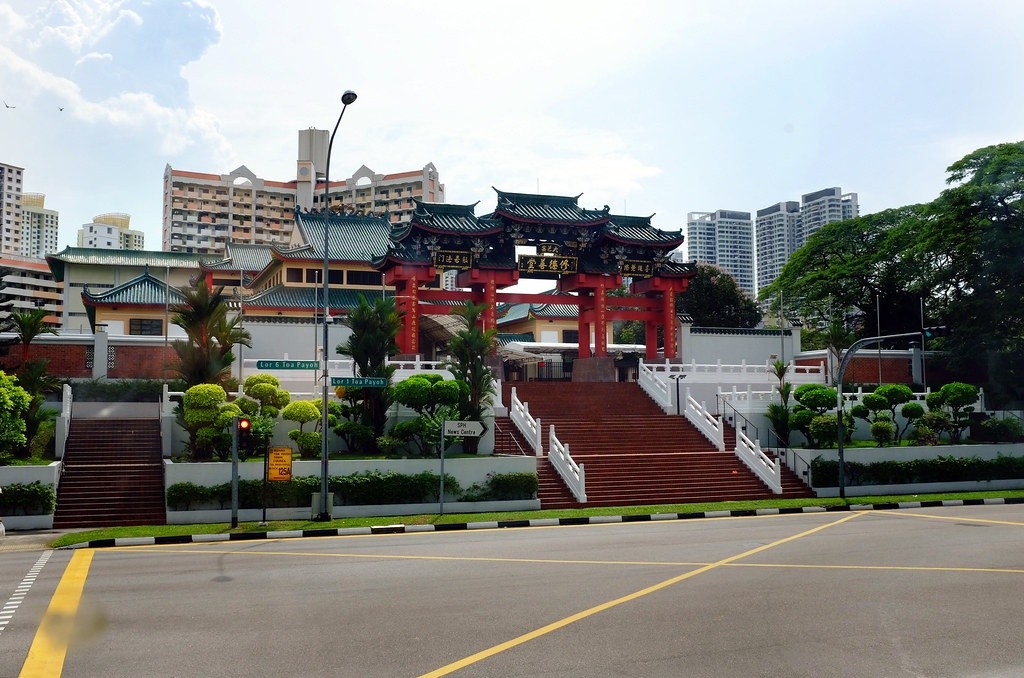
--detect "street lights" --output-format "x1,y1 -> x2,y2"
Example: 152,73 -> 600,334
315,88 -> 360,523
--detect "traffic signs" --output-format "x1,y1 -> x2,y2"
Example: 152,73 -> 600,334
444,420 -> 488,438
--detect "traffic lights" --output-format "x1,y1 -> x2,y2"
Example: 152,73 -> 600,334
921,325 -> 950,341
238,418 -> 252,448
